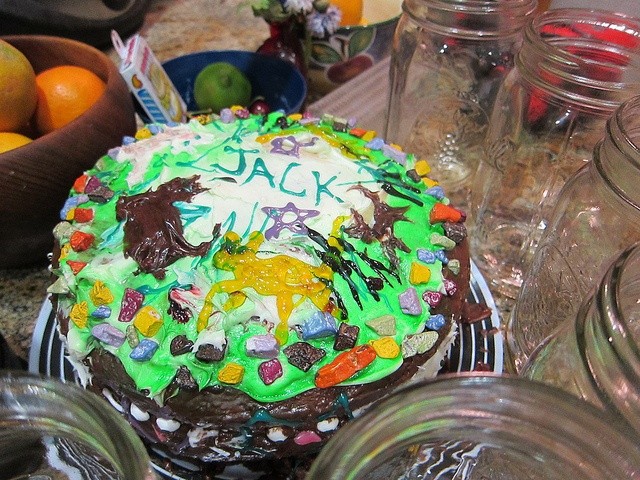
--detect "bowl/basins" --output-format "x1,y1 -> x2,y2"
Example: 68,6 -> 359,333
130,46 -> 311,127
0,34 -> 135,270
265,1 -> 411,101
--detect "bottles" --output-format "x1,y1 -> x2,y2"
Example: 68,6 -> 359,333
1,369 -> 155,480
505,97 -> 640,377
303,372 -> 640,480
519,242 -> 639,426
382,0 -> 539,192
462,6 -> 639,298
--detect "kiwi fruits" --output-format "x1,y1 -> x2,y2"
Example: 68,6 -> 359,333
194,63 -> 252,111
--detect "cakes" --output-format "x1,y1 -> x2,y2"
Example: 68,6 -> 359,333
29,104 -> 504,479
